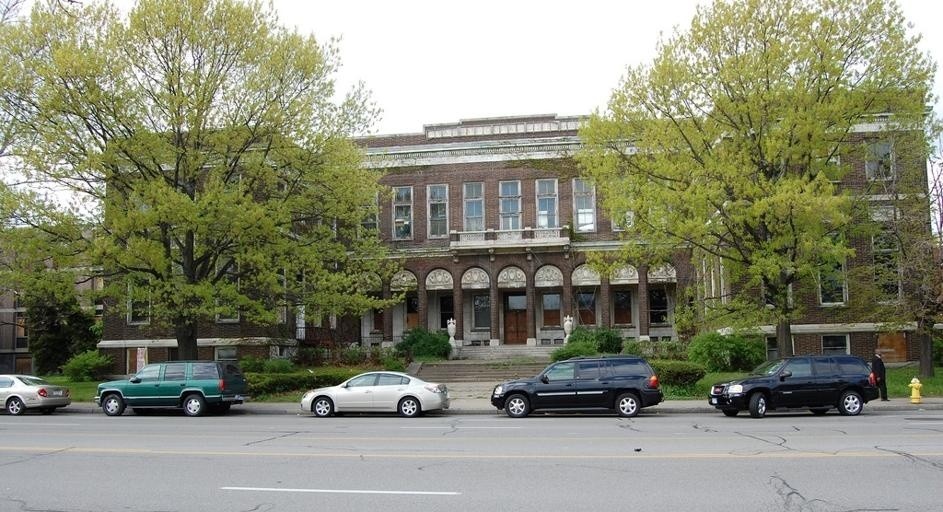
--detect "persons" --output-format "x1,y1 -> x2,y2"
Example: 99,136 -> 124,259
871,348 -> 891,402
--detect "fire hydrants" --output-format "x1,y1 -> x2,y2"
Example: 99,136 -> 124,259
906,376 -> 924,404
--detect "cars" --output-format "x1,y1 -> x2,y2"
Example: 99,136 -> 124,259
299,370 -> 451,418
0,373 -> 72,416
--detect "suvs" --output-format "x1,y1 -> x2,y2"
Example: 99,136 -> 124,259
93,358 -> 251,418
488,353 -> 667,419
707,353 -> 882,420
